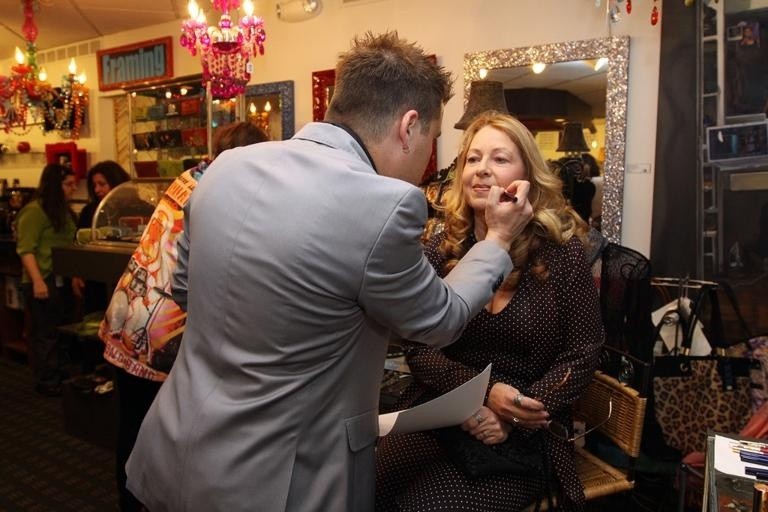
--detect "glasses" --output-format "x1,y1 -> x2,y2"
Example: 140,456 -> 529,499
536,368 -> 613,441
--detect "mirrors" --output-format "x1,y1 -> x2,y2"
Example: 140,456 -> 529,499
213,80 -> 296,147
312,55 -> 437,189
464,36 -> 629,249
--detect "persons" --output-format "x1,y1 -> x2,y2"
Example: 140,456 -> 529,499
377,107 -> 604,511
71,161 -> 157,374
15,163 -> 80,398
97,118 -> 270,512
126,31 -> 535,512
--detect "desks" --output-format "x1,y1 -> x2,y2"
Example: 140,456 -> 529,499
0,228 -> 141,396
698,429 -> 767,512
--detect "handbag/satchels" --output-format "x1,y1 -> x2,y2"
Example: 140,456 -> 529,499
652,337 -> 767,452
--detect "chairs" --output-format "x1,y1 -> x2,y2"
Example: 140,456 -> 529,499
527,370 -> 648,511
674,400 -> 767,512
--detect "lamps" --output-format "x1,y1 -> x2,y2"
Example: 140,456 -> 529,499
555,122 -> 590,153
176,1 -> 267,98
456,81 -> 509,132
0,0 -> 88,139
248,103 -> 273,130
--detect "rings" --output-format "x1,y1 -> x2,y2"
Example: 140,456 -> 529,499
481,431 -> 488,437
475,415 -> 483,424
514,393 -> 524,408
510,418 -> 520,428
